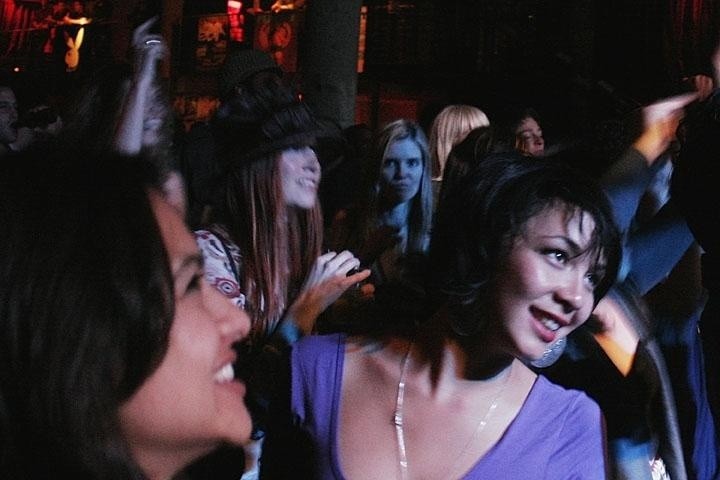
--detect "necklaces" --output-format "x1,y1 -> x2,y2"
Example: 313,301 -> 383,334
396,339 -> 512,479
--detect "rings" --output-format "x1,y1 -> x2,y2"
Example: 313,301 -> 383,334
144,39 -> 160,44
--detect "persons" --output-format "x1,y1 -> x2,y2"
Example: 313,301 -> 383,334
426,105 -> 544,211
0,170 -> 253,479
1,13 -> 167,169
191,104 -> 371,479
290,152 -> 620,479
598,75 -> 720,479
323,118 -> 436,335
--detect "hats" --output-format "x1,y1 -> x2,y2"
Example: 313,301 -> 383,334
230,99 -> 317,162
215,40 -> 282,101
